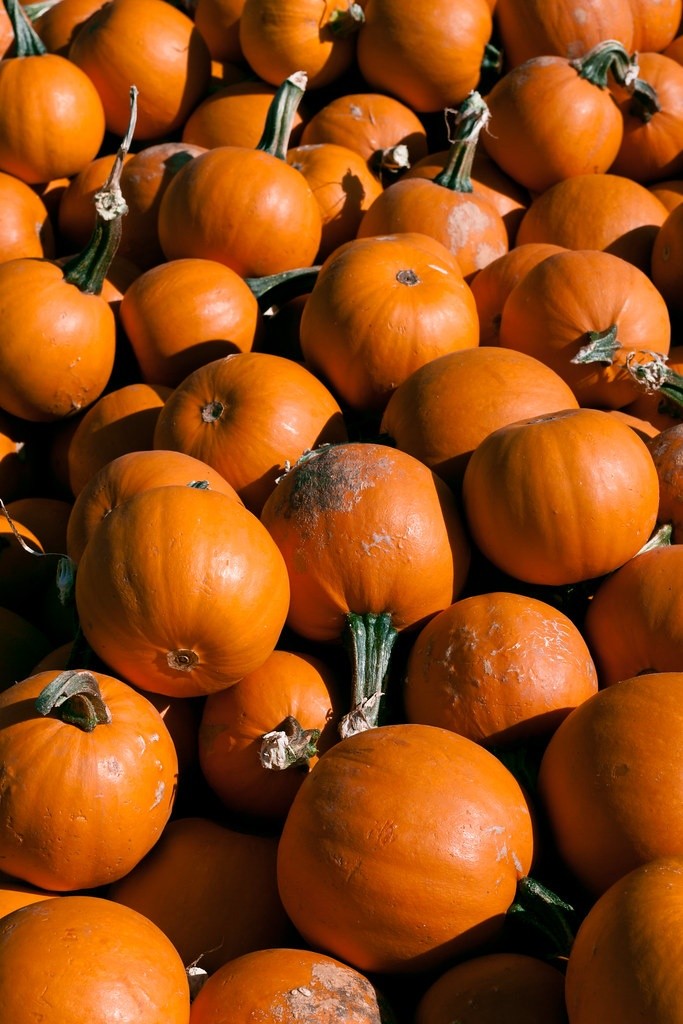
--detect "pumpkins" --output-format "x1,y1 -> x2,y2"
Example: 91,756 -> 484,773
0,0 -> 683,1024
